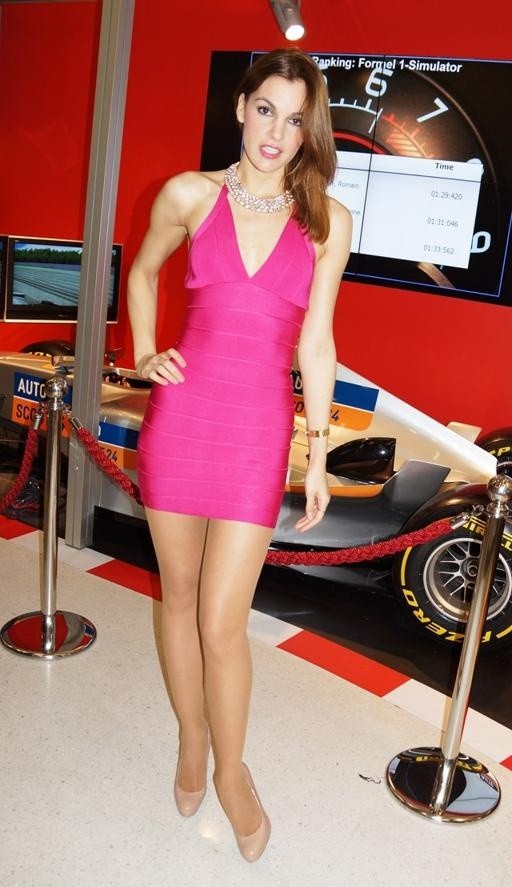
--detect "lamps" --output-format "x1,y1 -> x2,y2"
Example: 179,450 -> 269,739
268,0 -> 305,41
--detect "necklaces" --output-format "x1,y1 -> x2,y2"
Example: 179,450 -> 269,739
224,161 -> 295,215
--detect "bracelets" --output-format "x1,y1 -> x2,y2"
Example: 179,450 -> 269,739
303,427 -> 330,437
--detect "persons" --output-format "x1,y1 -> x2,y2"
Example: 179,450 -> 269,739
125,49 -> 355,864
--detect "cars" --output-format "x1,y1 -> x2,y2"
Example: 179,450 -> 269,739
1,340 -> 512,651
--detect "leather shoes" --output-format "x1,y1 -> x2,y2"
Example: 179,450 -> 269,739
175,720 -> 210,818
220,761 -> 271,862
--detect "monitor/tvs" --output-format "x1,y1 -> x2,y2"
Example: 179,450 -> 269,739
3,235 -> 123,325
198,48 -> 512,307
